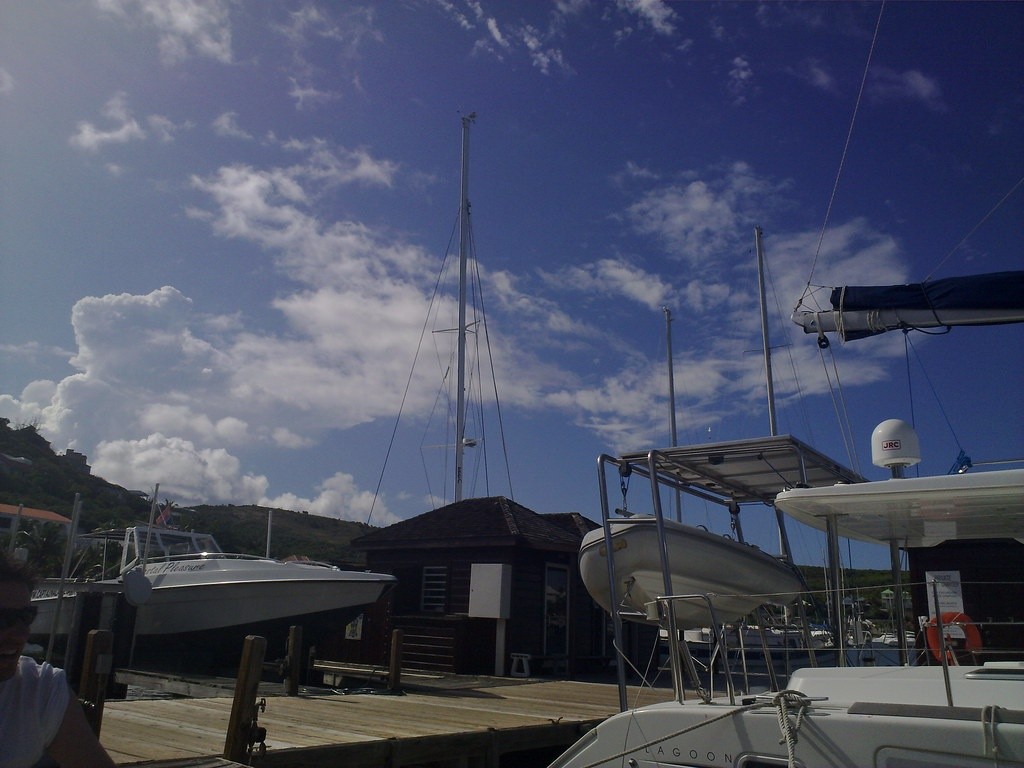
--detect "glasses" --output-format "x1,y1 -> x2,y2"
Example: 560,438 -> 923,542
0,606 -> 37,627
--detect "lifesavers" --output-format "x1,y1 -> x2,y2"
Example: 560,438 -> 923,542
926,611 -> 982,661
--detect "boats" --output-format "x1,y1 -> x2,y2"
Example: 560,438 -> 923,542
1,480 -> 398,648
517,262 -> 1024,768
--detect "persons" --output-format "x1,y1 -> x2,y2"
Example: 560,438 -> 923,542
0,547 -> 118,767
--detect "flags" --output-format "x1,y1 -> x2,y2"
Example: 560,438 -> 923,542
156,504 -> 171,528
